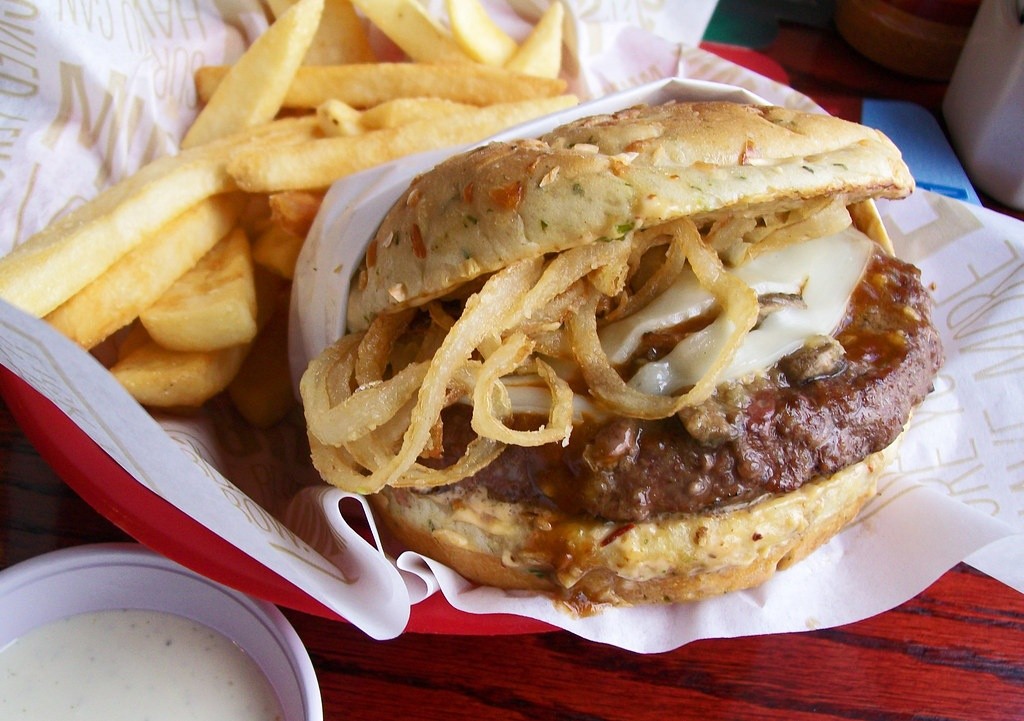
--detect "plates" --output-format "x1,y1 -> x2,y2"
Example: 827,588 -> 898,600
0,39 -> 781,636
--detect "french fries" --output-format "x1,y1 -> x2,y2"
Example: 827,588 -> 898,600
0,0 -> 580,431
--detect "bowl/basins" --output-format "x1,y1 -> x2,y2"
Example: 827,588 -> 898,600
0,540 -> 324,720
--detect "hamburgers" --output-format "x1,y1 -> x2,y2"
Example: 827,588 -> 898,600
297,101 -> 944,606
